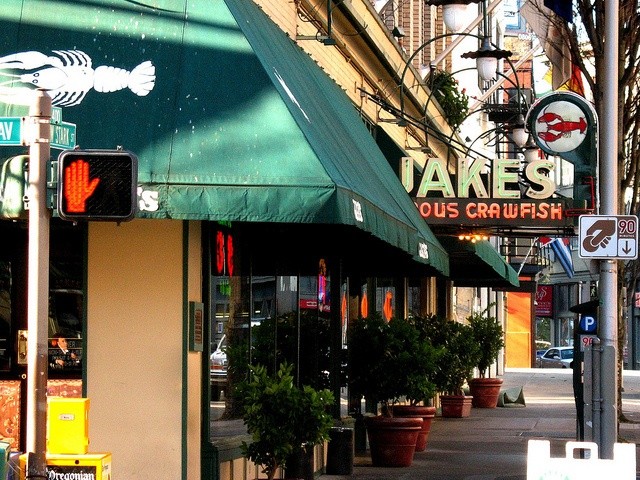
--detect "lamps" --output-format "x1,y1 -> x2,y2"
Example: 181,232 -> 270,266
465,126 -> 539,162
426,1 -> 479,34
400,32 -> 528,149
460,3 -> 511,82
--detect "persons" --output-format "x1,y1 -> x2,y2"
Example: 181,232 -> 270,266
50,338 -> 79,367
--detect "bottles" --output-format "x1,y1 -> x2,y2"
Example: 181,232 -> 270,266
382,400 -> 385,406
360,394 -> 367,415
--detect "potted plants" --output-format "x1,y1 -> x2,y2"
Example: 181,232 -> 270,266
349,310 -> 436,465
467,315 -> 504,408
439,319 -> 473,417
389,313 -> 443,453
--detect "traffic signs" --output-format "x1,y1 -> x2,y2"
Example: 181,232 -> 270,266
50,107 -> 63,125
49,121 -> 77,151
578,214 -> 638,259
0,117 -> 22,145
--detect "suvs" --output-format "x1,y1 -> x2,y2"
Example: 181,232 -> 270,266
210,333 -> 230,400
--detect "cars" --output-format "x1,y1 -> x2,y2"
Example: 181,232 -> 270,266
537,345 -> 575,368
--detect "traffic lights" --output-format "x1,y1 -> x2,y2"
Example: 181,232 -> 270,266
56,145 -> 137,222
